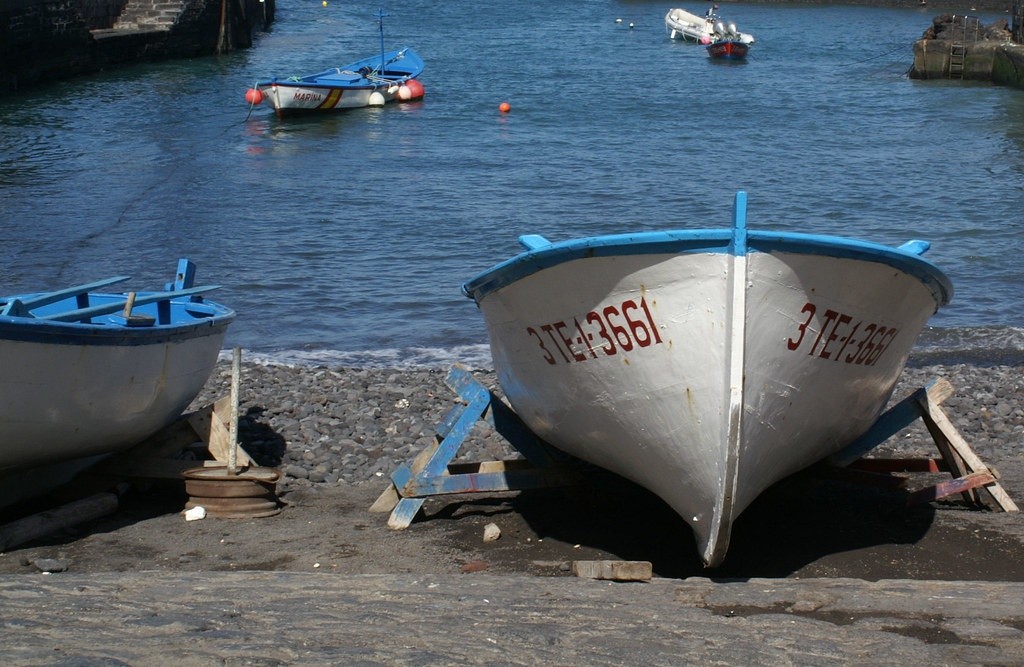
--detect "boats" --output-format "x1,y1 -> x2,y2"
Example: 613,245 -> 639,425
703,30 -> 750,62
664,4 -> 756,46
461,189 -> 956,573
252,7 -> 428,120
0,256 -> 240,509
